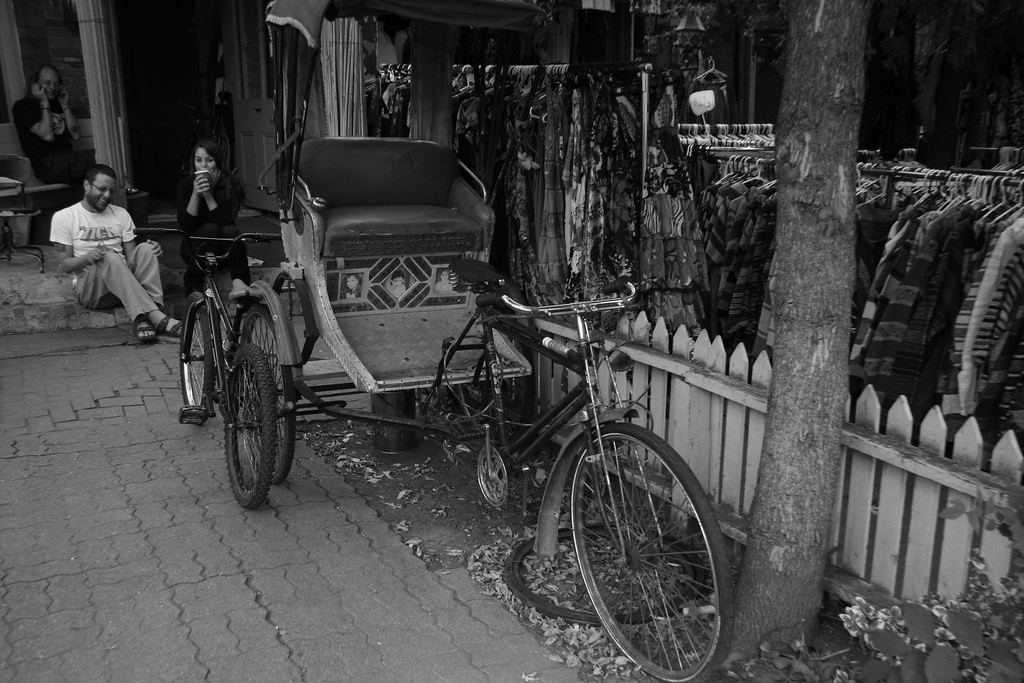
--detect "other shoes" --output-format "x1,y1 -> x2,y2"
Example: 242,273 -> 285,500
227,302 -> 248,317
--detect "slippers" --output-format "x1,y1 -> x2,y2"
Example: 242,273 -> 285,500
155,315 -> 183,336
134,317 -> 156,340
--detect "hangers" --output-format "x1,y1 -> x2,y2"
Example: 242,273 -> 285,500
678,122 -> 1024,224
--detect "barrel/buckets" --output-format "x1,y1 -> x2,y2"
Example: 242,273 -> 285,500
371,391 -> 418,455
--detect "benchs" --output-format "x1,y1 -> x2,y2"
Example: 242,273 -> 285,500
0,178 -> 71,197
278,137 -> 496,315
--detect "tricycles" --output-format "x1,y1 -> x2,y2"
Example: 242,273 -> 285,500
226,133 -> 733,683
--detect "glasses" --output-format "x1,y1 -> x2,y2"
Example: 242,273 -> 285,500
40,78 -> 63,88
89,182 -> 114,193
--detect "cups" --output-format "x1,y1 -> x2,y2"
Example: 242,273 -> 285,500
195,169 -> 210,184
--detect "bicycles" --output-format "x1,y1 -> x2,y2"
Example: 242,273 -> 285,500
134,226 -> 295,511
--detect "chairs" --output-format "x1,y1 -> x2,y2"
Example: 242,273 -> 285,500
0,154 -> 45,274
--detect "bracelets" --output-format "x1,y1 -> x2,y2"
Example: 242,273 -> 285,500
41,108 -> 51,110
63,105 -> 69,110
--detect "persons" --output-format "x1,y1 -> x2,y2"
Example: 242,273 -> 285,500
49,164 -> 184,341
177,140 -> 251,292
12,64 -> 138,195
